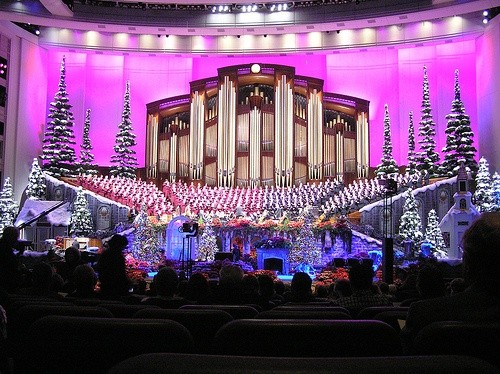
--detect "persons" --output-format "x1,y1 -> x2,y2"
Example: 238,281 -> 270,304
0,210 -> 500,339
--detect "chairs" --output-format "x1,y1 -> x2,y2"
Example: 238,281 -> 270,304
0,294 -> 500,374
